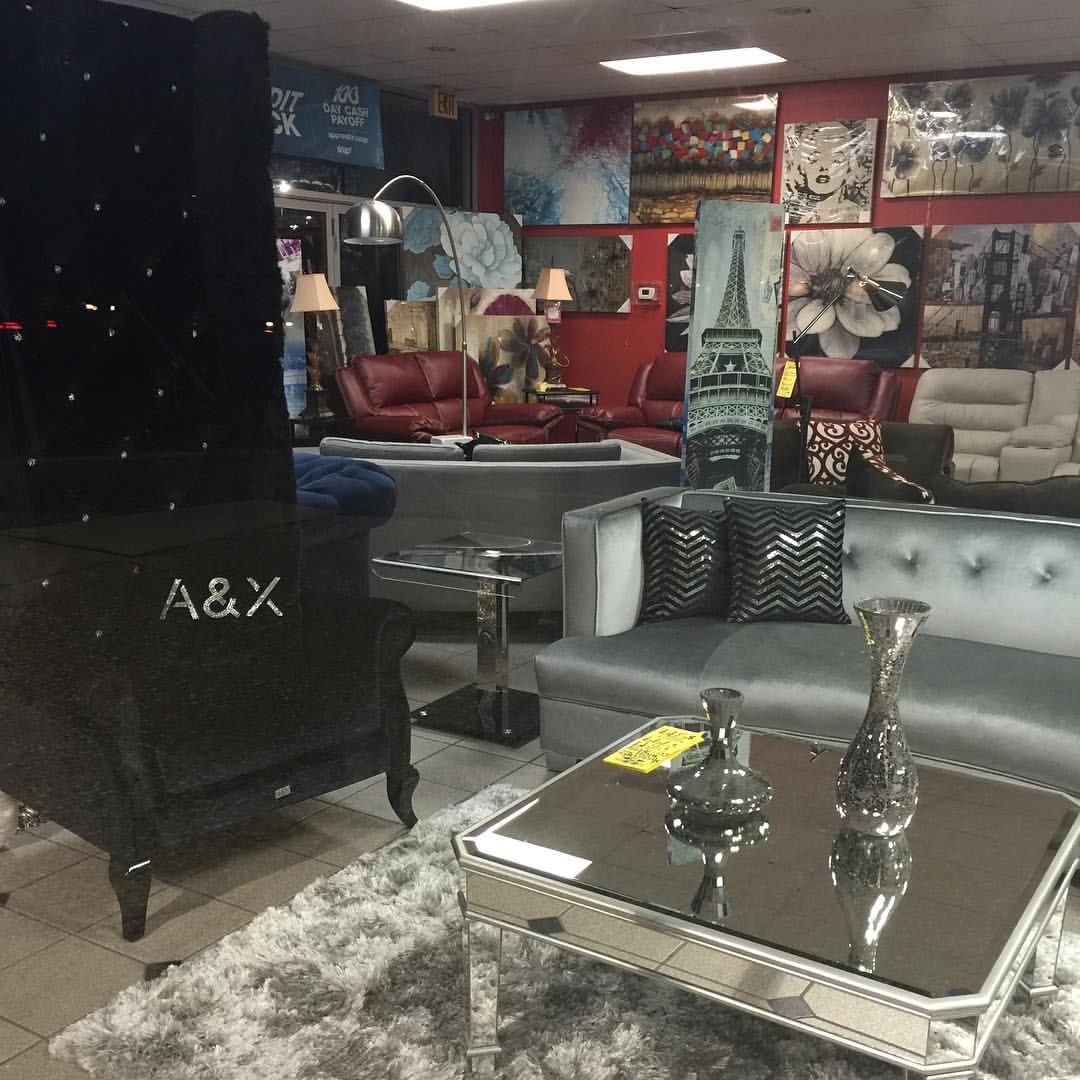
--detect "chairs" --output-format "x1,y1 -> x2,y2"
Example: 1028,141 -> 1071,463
0,0 -> 419,942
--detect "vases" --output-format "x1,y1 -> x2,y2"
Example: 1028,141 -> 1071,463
832,826 -> 913,978
663,802 -> 767,923
666,687 -> 775,815
836,597 -> 932,836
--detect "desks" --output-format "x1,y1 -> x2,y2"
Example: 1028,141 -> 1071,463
372,531 -> 563,748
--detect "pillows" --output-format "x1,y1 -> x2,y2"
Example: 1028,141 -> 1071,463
638,497 -> 853,625
846,437 -> 934,504
795,418 -> 885,485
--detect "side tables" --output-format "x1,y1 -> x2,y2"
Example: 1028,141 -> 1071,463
289,416 -> 354,446
522,386 -> 599,408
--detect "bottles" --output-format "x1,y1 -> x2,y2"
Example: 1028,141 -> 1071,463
665,804 -> 772,921
665,687 -> 775,814
829,828 -> 913,974
835,596 -> 934,837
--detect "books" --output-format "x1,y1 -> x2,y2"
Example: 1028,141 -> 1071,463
536,381 -> 598,404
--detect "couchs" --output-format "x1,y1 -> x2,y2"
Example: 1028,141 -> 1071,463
337,349 -> 565,444
908,368 -> 1080,483
291,438 -> 681,612
576,352 -> 895,458
771,417 -> 1080,519
533,486 -> 1080,871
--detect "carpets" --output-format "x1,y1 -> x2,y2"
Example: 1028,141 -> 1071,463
48,784 -> 1080,1080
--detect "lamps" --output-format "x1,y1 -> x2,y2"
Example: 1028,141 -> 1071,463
529,266 -> 573,389
289,260 -> 340,418
342,174 -> 472,446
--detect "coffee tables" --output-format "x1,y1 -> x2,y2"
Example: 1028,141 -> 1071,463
451,716 -> 1080,1080
